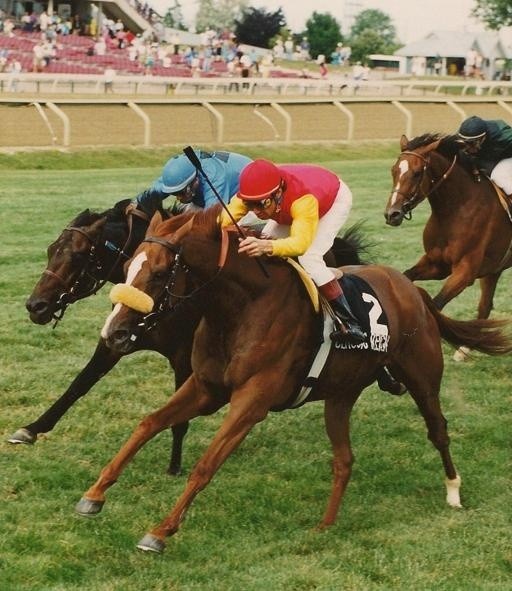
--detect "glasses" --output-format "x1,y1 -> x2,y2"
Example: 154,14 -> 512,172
244,195 -> 275,210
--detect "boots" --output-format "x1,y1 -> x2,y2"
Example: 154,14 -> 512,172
319,278 -> 367,344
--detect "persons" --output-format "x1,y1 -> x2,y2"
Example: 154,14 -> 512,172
130,149 -> 256,224
352,60 -> 371,80
0,0 -> 273,77
103,65 -> 115,94
458,117 -> 512,199
410,56 -> 426,77
217,156 -> 371,342
466,46 -> 483,79
433,54 -> 443,76
274,37 -> 310,60
338,46 -> 352,65
319,61 -> 328,80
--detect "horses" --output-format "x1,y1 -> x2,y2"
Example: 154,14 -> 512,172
382,132 -> 512,362
4,184 -> 512,557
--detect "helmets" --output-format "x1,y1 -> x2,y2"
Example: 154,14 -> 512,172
236,158 -> 283,202
458,116 -> 489,141
158,153 -> 199,194
